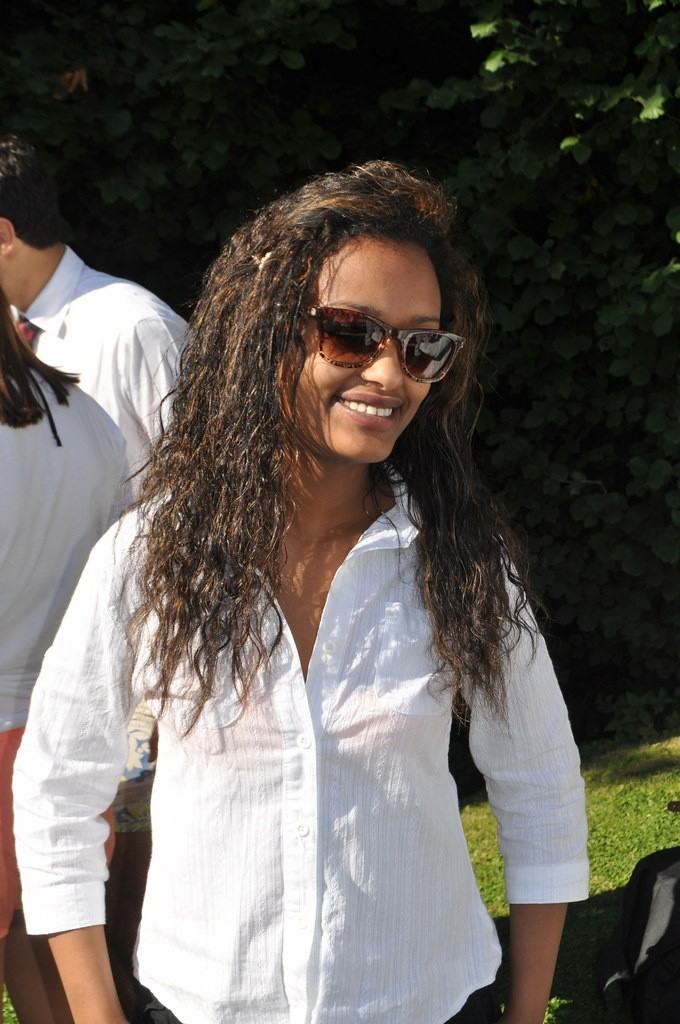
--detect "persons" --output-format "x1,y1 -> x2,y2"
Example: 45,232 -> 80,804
10,161 -> 588,1024
0,295 -> 138,1024
0,135 -> 207,1024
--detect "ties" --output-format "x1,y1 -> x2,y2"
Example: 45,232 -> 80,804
16,316 -> 39,350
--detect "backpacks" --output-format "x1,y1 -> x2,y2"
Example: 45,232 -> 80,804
602,845 -> 680,1024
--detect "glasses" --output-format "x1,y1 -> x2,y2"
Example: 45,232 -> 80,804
300,304 -> 466,383
